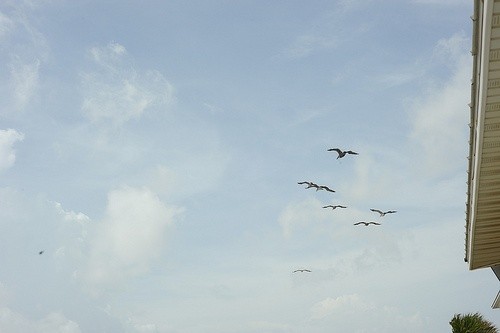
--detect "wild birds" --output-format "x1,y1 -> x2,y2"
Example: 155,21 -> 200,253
328,148 -> 359,160
323,205 -> 347,210
293,269 -> 312,273
354,221 -> 381,226
298,181 -> 335,192
371,209 -> 397,217
